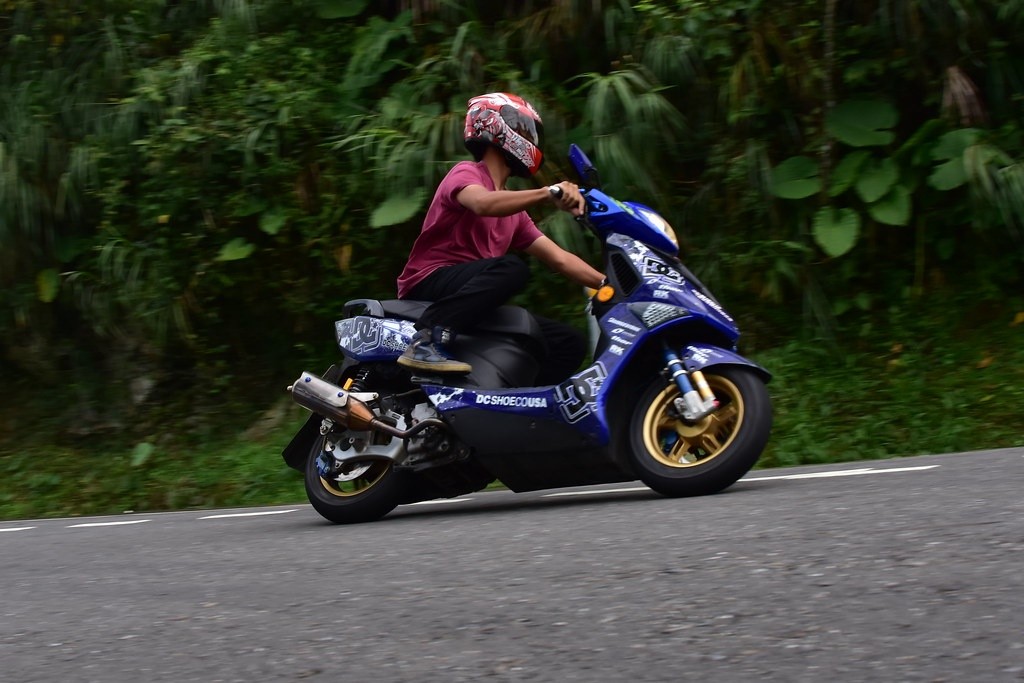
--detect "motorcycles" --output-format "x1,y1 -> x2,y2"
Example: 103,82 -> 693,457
280,144 -> 773,526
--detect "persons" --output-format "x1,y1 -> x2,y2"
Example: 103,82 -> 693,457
397,91 -> 610,378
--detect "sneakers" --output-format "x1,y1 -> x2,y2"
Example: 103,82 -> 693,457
397,328 -> 471,372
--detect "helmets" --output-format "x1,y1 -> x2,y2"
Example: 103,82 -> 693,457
464,92 -> 544,177
